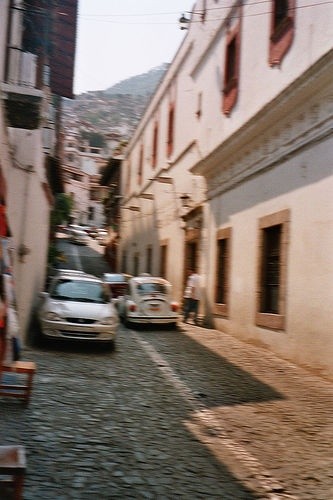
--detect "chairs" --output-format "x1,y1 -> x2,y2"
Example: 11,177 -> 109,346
0,316 -> 36,403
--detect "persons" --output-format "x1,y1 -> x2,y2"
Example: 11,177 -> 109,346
181,266 -> 203,323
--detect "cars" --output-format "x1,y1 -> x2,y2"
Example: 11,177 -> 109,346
101,273 -> 133,297
117,276 -> 180,329
55,223 -> 110,246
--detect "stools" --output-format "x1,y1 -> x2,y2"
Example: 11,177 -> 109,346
0,446 -> 27,500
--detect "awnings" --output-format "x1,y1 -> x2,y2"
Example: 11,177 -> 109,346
23,0 -> 79,101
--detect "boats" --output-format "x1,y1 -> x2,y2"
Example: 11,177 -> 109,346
38,275 -> 122,347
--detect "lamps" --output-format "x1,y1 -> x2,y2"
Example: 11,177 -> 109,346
180,193 -> 194,209
